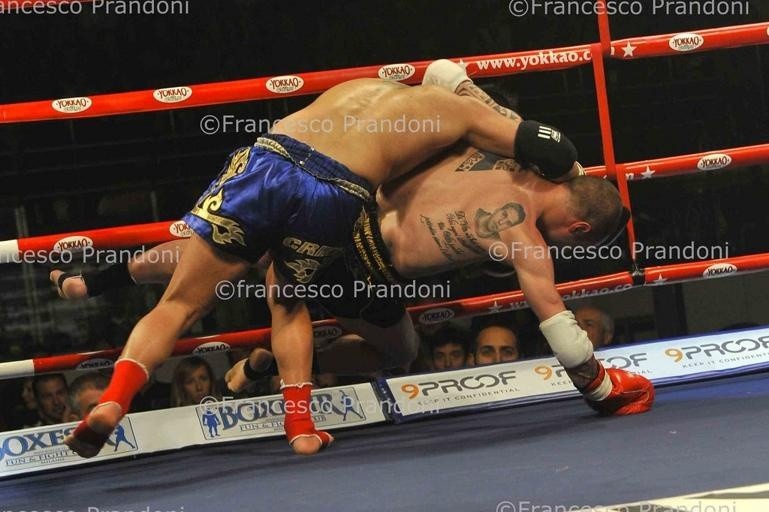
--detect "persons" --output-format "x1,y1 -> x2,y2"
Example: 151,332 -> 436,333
19,301 -> 615,430
59,77 -> 586,458
473,201 -> 527,243
48,146 -> 654,416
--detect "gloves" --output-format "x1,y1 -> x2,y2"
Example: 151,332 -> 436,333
583,361 -> 655,415
